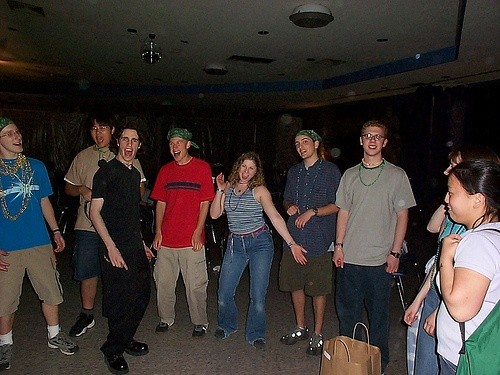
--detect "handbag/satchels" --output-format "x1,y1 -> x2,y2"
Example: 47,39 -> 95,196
455,300 -> 500,375
319,323 -> 382,375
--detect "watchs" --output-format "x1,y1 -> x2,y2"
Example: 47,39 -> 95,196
312,207 -> 319,217
390,251 -> 401,259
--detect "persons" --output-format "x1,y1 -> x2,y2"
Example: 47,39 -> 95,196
436,157 -> 500,375
333,120 -> 409,374
64,111 -> 145,337
89,124 -> 155,375
403,143 -> 492,375
153,129 -> 213,338
0,115 -> 80,371
280,130 -> 342,356
210,151 -> 308,349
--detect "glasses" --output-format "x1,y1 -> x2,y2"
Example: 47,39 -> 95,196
362,132 -> 385,140
0,129 -> 23,138
92,126 -> 109,131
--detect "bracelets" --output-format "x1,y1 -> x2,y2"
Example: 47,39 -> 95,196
216,188 -> 224,193
335,242 -> 344,248
52,228 -> 60,234
286,241 -> 296,247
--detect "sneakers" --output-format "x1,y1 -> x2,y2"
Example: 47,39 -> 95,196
102,350 -> 129,375
0,344 -> 13,369
306,333 -> 324,355
192,321 -> 209,339
280,327 -> 310,345
122,341 -> 149,356
47,328 -> 78,355
215,329 -> 226,339
154,321 -> 175,334
69,312 -> 95,337
254,339 -> 266,350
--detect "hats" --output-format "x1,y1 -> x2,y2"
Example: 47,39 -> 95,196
0,118 -> 14,131
168,129 -> 199,150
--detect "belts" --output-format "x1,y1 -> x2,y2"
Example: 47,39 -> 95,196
236,226 -> 267,237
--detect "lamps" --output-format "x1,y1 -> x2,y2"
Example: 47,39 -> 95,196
288,3 -> 334,29
140,41 -> 161,65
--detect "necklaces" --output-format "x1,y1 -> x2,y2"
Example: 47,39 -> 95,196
94,144 -> 112,163
358,157 -> 386,186
228,178 -> 249,211
295,155 -> 324,232
429,221 -> 451,291
0,153 -> 34,220
178,156 -> 192,165
115,155 -> 133,170
